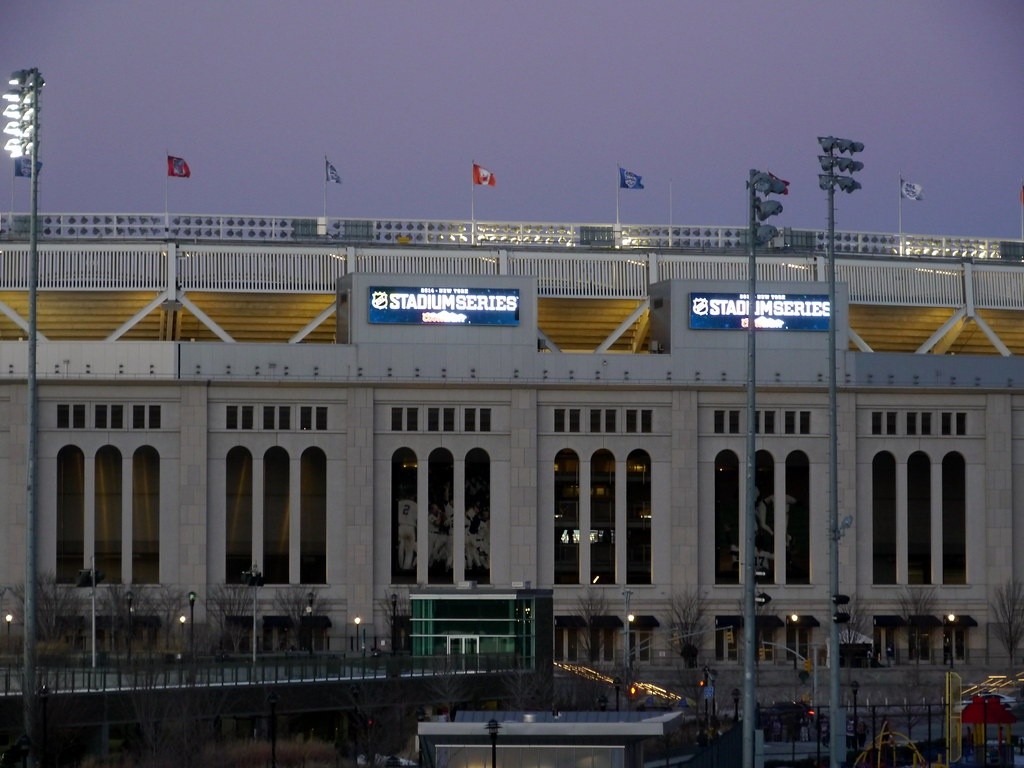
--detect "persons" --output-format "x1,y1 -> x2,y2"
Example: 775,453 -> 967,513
398,493 -> 489,569
219,631 -> 249,652
768,714 -> 869,750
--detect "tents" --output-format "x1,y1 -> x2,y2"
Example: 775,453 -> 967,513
825,629 -> 873,667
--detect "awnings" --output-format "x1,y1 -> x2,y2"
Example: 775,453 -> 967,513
717,616 -> 744,626
944,616 -> 977,626
755,616 -> 784,627
634,616 -> 659,627
909,615 -> 942,626
226,616 -> 331,628
873,616 -> 907,628
592,616 -> 623,627
54,615 -> 161,628
554,615 -> 586,626
787,617 -> 820,627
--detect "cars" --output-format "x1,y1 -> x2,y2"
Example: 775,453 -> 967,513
762,701 -> 816,726
953,691 -> 1019,714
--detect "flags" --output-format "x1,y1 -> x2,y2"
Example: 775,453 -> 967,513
168,156 -> 190,177
472,164 -> 495,186
901,180 -> 924,200
620,168 -> 644,188
14,157 -> 42,177
326,160 -> 340,183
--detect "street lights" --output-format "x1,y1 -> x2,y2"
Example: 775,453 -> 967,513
815,135 -> 865,768
484,718 -> 503,768
627,614 -> 635,669
306,592 -> 315,652
850,679 -> 859,751
126,591 -> 135,659
240,563 -> 262,682
612,676 -> 622,712
355,617 -> 360,653
188,590 -> 196,660
947,614 -> 960,669
6,613 -> 14,691
740,167 -> 791,768
75,555 -> 105,690
790,614 -> 799,670
2,65 -> 46,768
